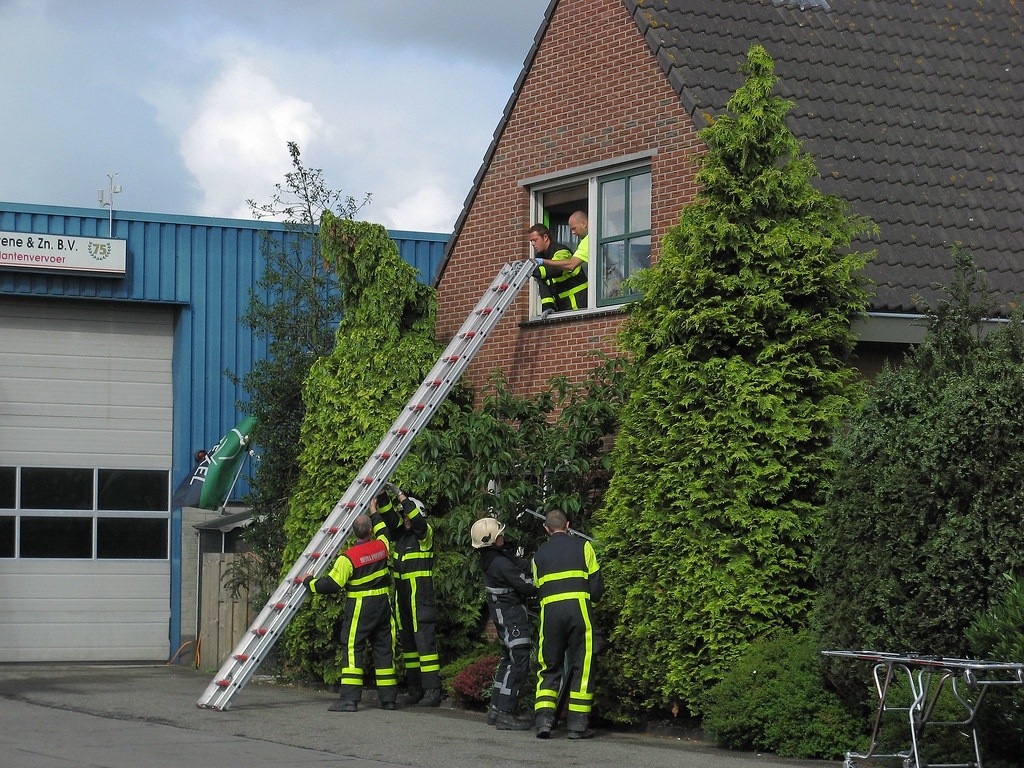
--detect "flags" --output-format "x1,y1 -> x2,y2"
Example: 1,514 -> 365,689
172,414 -> 257,511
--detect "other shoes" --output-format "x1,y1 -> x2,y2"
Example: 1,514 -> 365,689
400,691 -> 422,706
416,693 -> 443,709
535,724 -> 552,737
327,700 -> 359,711
376,702 -> 398,711
486,707 -> 501,726
495,712 -> 532,731
568,728 -> 597,738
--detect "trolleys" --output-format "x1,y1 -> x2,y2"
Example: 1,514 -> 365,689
819,648 -> 1024,768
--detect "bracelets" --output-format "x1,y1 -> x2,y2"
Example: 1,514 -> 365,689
398,491 -> 404,496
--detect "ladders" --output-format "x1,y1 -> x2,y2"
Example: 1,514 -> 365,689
194,254 -> 537,712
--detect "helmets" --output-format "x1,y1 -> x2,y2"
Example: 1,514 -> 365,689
396,496 -> 428,519
470,518 -> 506,549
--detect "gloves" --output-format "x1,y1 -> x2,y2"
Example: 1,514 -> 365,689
515,262 -> 525,272
385,481 -> 402,496
541,308 -> 556,317
533,257 -> 545,267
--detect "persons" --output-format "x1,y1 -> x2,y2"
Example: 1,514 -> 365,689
470,518 -> 534,730
378,481 -> 443,707
515,210 -> 643,320
302,499 -> 397,712
531,509 -> 604,739
